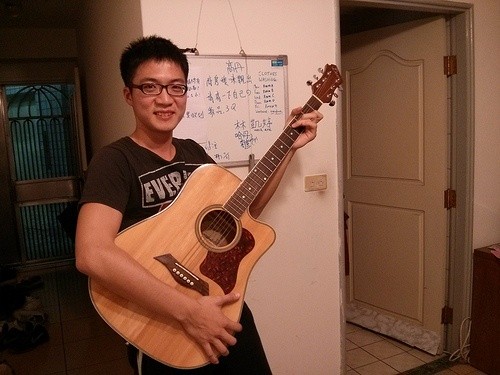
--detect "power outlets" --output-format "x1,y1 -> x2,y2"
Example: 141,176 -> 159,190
302,174 -> 329,192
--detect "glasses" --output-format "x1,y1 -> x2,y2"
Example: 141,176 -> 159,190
129,80 -> 191,99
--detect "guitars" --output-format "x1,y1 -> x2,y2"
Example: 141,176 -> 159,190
87,62 -> 344,369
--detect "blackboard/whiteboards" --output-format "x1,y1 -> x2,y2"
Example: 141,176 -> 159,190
171,54 -> 287,168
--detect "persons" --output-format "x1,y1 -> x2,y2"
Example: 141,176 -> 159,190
74,34 -> 324,375
